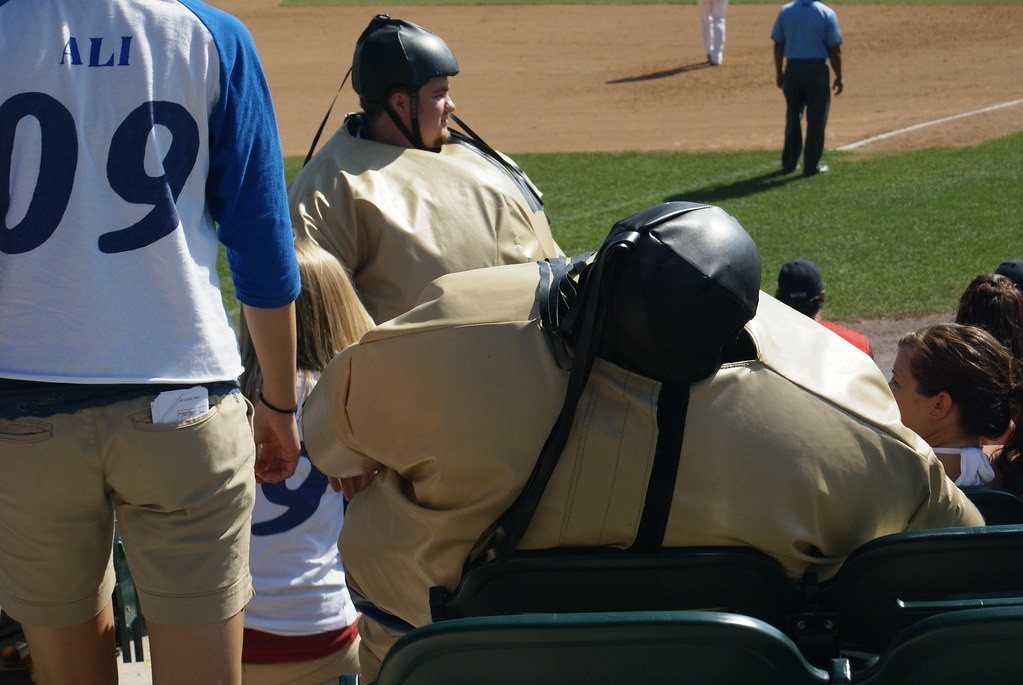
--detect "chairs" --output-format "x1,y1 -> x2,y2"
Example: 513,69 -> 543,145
337,486 -> 1023,685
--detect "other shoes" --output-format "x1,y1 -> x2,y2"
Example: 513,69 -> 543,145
817,164 -> 829,175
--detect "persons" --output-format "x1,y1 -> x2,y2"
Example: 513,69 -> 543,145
299,204 -> 988,685
770,0 -> 844,176
238,243 -> 380,685
774,258 -> 1023,496
0,0 -> 303,685
289,12 -> 565,326
699,0 -> 727,66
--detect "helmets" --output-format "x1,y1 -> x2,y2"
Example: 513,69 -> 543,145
351,14 -> 460,102
574,200 -> 760,382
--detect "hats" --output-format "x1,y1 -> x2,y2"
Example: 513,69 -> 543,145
775,260 -> 823,313
995,260 -> 1023,291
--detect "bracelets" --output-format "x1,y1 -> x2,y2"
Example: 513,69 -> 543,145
258,391 -> 299,415
